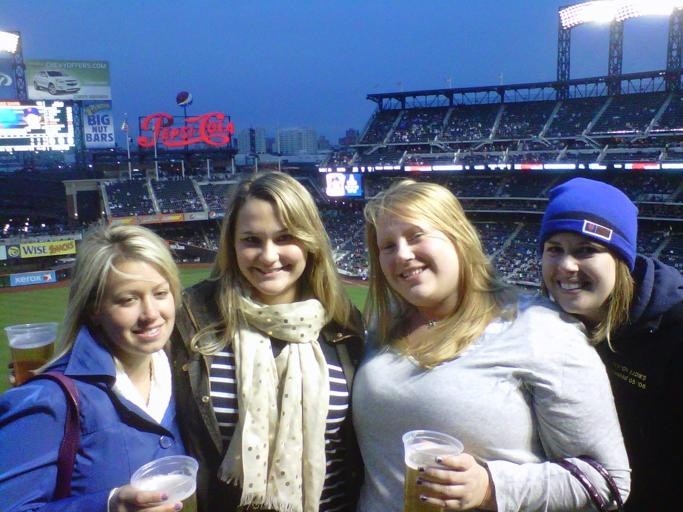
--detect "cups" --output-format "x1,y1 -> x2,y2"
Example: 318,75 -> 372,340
401,429 -> 465,511
6,320 -> 58,391
131,455 -> 200,510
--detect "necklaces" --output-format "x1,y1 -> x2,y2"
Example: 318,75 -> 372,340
420,318 -> 449,327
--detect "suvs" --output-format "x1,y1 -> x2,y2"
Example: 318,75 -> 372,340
31,70 -> 81,95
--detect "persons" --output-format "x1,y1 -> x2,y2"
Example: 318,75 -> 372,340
7,171 -> 365,512
530,176 -> 683,512
350,183 -> 632,512
1,226 -> 200,512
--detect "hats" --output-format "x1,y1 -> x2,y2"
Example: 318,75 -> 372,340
537,177 -> 640,273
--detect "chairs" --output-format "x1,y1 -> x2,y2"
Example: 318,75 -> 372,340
328,172 -> 418,281
591,171 -> 680,218
637,220 -> 681,270
420,172 -> 552,210
470,213 -> 543,287
102,165 -> 327,262
344,93 -> 681,163
